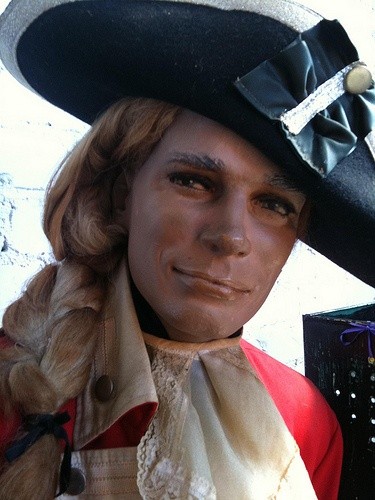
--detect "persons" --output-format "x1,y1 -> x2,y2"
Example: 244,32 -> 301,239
0,0 -> 375,500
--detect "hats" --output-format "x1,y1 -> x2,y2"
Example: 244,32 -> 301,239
1,0 -> 375,288
